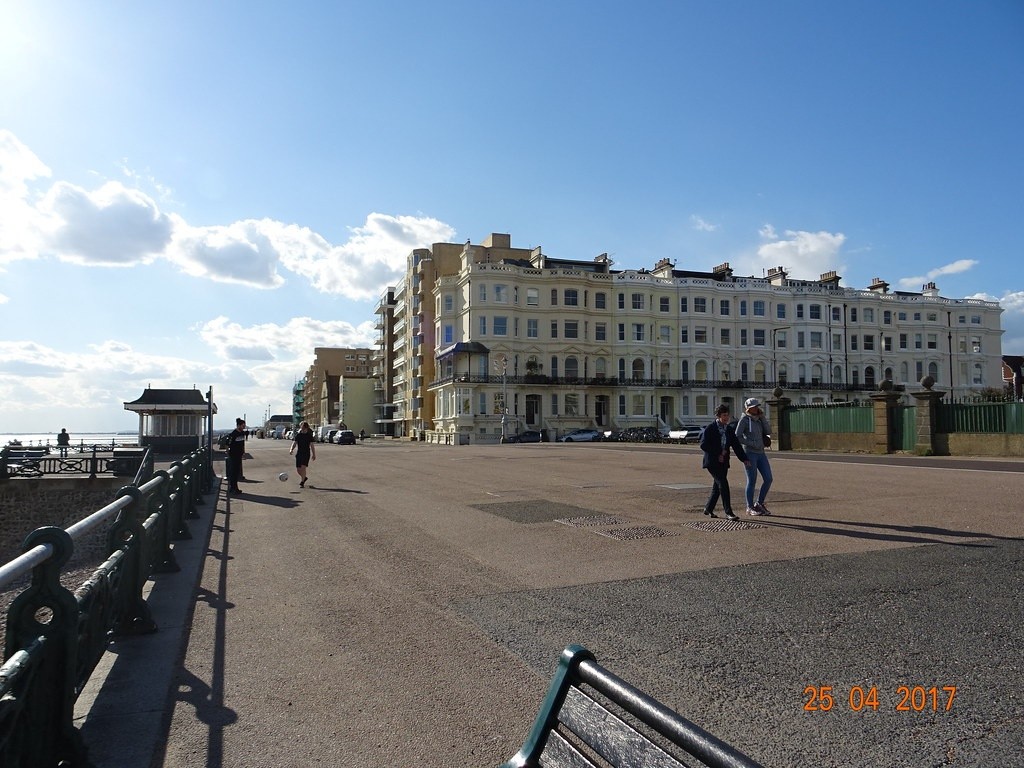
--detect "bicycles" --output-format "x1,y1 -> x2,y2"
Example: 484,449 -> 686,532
602,425 -> 704,445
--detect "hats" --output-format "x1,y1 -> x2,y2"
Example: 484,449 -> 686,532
744,398 -> 761,409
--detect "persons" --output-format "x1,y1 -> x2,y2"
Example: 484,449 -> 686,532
289,421 -> 316,488
735,398 -> 773,516
225,418 -> 246,494
360,428 -> 365,437
700,404 -> 751,521
58,429 -> 69,457
218,432 -> 226,446
245,429 -> 257,441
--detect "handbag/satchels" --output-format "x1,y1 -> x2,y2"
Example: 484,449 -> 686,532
759,418 -> 771,447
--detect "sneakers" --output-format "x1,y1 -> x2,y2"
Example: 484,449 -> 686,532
746,507 -> 759,516
754,502 -> 771,515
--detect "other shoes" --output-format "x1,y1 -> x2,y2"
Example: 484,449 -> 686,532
726,514 -> 739,520
704,508 -> 719,518
300,477 -> 308,488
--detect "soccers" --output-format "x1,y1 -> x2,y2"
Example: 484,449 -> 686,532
279,472 -> 288,482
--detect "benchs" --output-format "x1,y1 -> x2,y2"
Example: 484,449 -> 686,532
492,644 -> 769,768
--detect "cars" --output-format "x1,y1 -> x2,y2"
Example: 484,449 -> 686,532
267,425 -> 294,440
509,431 -> 549,443
556,429 -> 601,443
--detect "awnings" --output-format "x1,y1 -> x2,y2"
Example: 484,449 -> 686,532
435,342 -> 490,360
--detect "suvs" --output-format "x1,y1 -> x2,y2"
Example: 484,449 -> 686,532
332,429 -> 356,445
323,429 -> 337,443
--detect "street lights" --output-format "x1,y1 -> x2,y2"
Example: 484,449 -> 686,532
773,326 -> 791,389
880,331 -> 883,381
501,355 -> 509,443
263,404 -> 270,425
828,303 -> 832,383
